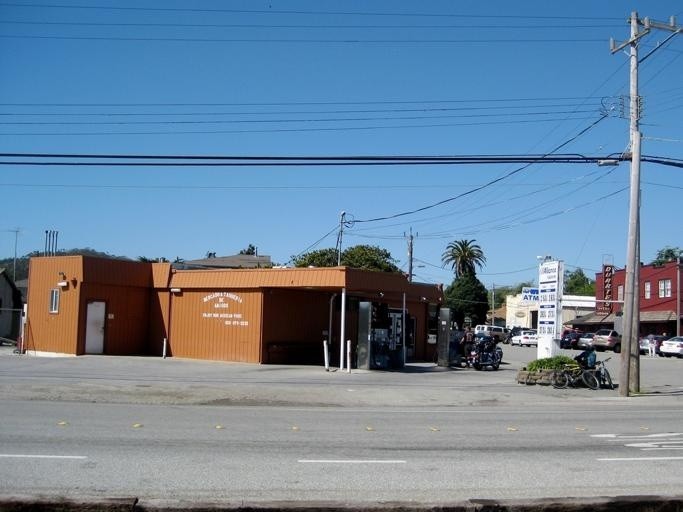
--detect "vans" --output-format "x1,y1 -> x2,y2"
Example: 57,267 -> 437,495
475,325 -> 509,343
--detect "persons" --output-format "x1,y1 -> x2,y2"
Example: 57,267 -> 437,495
459,326 -> 474,357
574,342 -> 596,368
647,337 -> 656,357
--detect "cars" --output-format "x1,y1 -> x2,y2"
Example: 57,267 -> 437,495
560,329 -> 622,353
472,334 -> 503,358
510,330 -> 537,347
660,336 -> 683,357
640,335 -> 670,355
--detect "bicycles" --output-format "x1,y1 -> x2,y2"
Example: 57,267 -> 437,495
550,364 -> 600,391
593,358 -> 614,390
